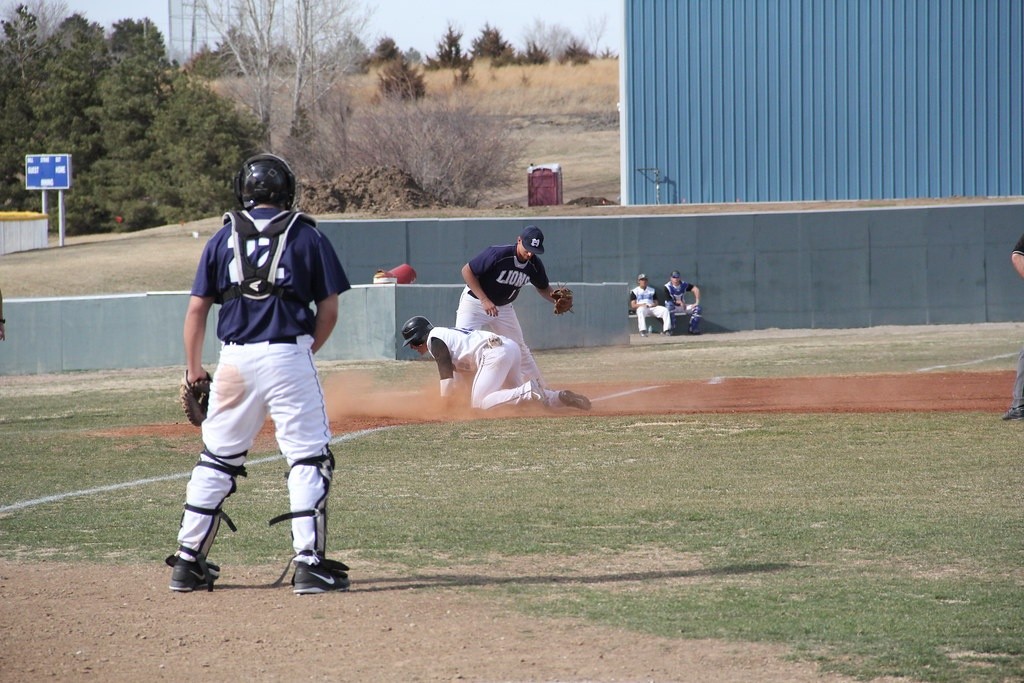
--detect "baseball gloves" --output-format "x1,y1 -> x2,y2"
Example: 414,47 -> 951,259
549,280 -> 576,316
179,367 -> 212,428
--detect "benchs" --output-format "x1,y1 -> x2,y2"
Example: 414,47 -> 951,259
628,311 -> 693,334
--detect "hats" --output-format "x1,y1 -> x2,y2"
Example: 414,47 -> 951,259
521,225 -> 544,254
637,274 -> 649,280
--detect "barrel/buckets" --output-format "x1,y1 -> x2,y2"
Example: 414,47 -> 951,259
388,263 -> 416,284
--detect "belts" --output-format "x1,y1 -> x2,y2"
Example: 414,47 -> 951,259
468,290 -> 479,299
224,335 -> 297,345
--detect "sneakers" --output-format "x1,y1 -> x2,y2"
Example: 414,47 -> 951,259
169,563 -> 216,590
293,561 -> 350,593
559,390 -> 592,411
1001,406 -> 1024,421
531,378 -> 549,408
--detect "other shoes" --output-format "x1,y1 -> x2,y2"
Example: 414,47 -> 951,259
665,330 -> 672,336
688,327 -> 701,335
671,329 -> 678,335
640,330 -> 648,337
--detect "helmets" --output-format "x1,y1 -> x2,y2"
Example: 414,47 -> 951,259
234,153 -> 295,209
401,316 -> 434,347
671,271 -> 680,278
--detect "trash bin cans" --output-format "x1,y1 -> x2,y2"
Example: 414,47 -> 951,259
528,164 -> 564,205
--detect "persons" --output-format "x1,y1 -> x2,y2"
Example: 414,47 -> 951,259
452,223 -> 572,389
1001,234 -> 1024,420
664,270 -> 704,334
164,154 -> 353,595
401,317 -> 592,412
630,274 -> 672,337
